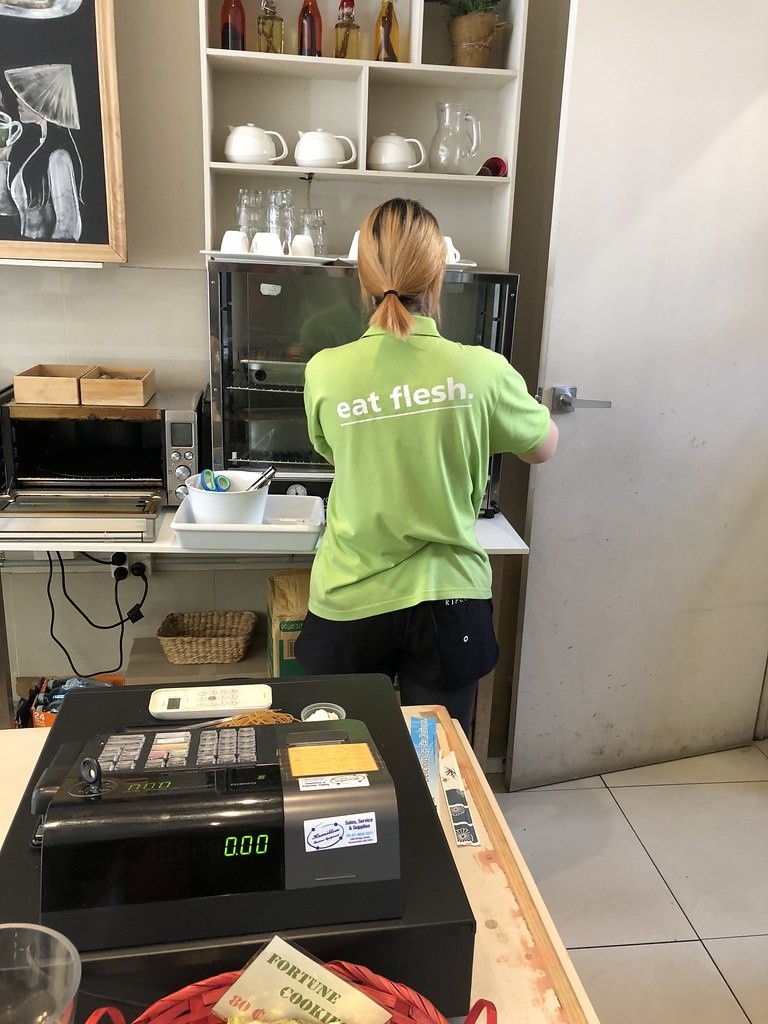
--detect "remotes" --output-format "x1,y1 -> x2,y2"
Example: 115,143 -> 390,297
147,684 -> 273,720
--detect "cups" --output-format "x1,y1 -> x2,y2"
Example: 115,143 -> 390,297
300,702 -> 346,722
233,188 -> 264,241
265,187 -> 295,252
221,230 -> 249,254
288,235 -> 315,257
347,230 -> 361,260
0,922 -> 82,1024
443,236 -> 461,264
249,232 -> 285,256
299,209 -> 328,256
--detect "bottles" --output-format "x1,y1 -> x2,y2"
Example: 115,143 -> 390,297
374,0 -> 401,63
255,0 -> 285,54
333,0 -> 361,59
297,0 -> 323,57
220,0 -> 246,51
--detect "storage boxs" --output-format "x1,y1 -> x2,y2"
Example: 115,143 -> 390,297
80,366 -> 156,406
13,364 -> 98,404
265,569 -> 313,678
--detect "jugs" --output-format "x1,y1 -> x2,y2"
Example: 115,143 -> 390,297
293,128 -> 359,169
224,122 -> 288,165
429,101 -> 481,175
367,133 -> 427,172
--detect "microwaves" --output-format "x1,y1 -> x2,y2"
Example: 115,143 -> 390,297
0,384 -> 213,508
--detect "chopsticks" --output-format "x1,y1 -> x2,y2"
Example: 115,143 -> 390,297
179,708 -> 283,729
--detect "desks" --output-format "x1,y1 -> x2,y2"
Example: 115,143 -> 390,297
1,509 -> 530,777
0,717 -> 600,1024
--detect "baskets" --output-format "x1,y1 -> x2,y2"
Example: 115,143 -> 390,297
157,610 -> 256,665
83,960 -> 498,1024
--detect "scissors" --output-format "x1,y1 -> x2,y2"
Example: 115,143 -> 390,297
201,469 -> 231,492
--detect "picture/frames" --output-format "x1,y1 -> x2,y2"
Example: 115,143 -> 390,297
0,0 -> 129,265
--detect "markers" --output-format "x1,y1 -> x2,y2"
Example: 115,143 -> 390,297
247,466 -> 277,491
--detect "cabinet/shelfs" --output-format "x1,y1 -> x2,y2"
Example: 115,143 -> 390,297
201,0 -> 529,270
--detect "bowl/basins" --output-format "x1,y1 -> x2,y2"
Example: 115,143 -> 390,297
186,470 -> 272,525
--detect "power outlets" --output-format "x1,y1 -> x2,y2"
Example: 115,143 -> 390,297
110,552 -> 152,579
107,552 -> 152,578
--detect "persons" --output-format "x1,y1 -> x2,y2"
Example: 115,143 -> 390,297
294,198 -> 560,739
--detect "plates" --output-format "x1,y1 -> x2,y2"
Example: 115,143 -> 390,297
200,249 -> 340,267
338,255 -> 358,267
447,259 -> 480,273
